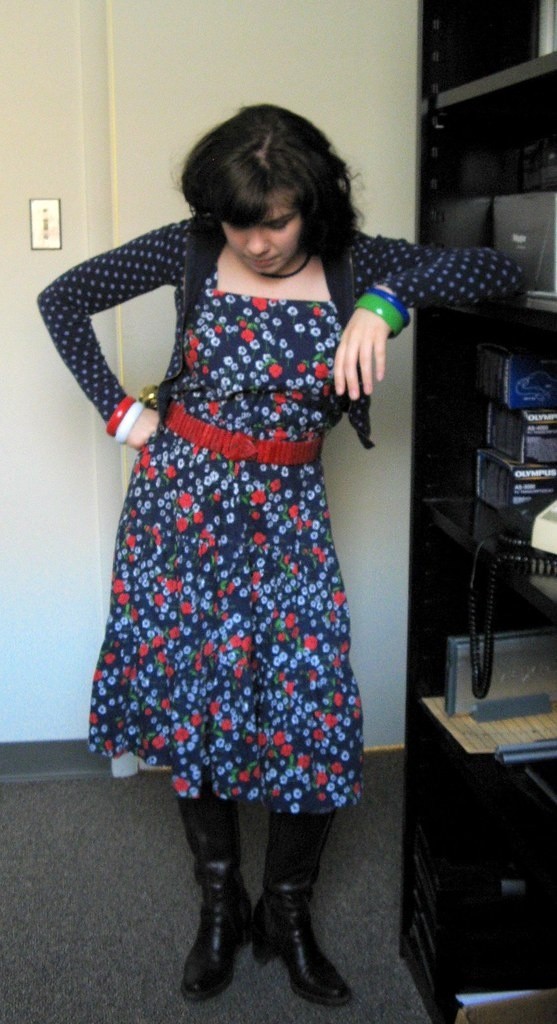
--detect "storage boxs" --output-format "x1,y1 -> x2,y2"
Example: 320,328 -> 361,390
469,340 -> 557,511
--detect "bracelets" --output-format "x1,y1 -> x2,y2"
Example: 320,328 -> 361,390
107,396 -> 144,442
353,288 -> 410,338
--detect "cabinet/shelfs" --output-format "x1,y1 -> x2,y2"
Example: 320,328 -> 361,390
397,0 -> 557,1024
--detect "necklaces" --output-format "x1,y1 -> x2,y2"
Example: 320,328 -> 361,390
260,252 -> 312,279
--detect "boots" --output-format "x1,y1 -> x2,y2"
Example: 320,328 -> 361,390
180,797 -> 254,999
251,808 -> 353,1006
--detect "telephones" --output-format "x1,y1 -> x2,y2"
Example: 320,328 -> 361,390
503,488 -> 557,565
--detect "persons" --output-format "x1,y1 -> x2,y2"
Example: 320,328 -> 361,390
38,104 -> 523,1004
